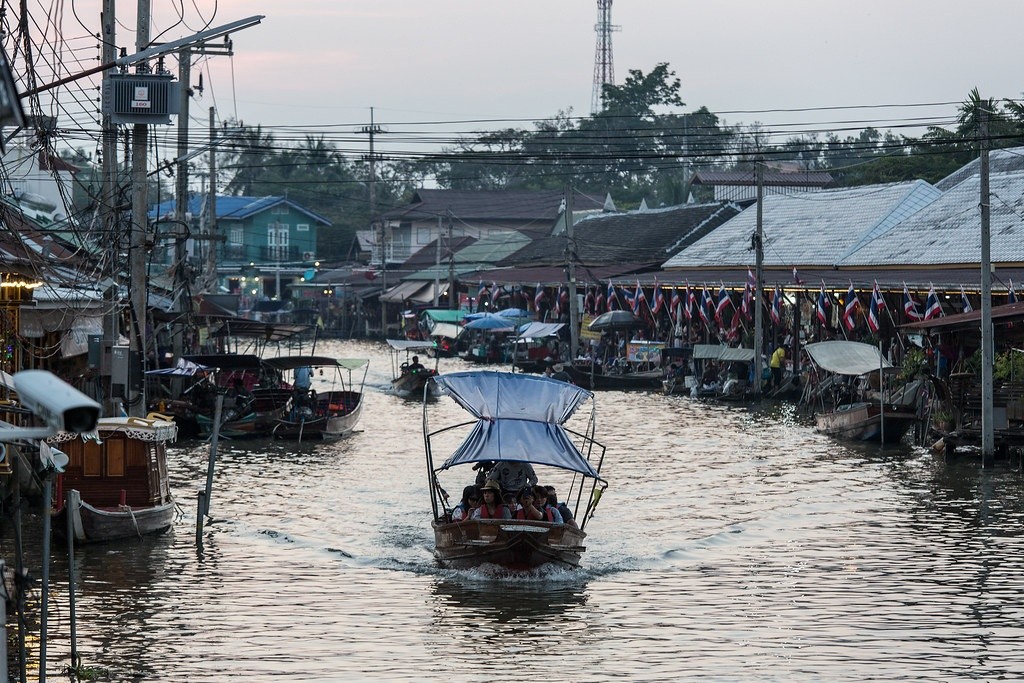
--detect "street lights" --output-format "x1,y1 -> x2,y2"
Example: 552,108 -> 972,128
230,261 -> 260,310
313,261 -> 320,305
323,280 -> 333,332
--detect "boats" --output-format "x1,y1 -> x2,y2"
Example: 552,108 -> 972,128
35,413 -> 178,542
401,309 -> 772,398
260,355 -> 370,442
386,338 -> 442,401
804,340 -> 893,443
190,313 -> 318,439
422,369 -> 608,573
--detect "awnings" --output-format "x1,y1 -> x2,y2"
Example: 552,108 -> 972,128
379,281 -> 450,304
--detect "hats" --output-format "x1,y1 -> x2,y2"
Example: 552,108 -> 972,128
479,479 -> 500,490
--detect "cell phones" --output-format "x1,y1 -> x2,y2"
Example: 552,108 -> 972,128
523,487 -> 531,500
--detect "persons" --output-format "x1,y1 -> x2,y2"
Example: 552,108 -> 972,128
294,366 -> 315,390
583,325 -> 952,378
408,356 -> 424,371
452,459 -> 578,529
771,345 -> 789,396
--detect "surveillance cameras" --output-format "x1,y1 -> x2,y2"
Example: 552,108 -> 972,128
12,370 -> 102,433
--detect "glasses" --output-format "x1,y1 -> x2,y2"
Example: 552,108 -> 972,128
468,496 -> 479,504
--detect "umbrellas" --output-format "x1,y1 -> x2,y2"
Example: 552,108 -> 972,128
587,310 -> 650,330
464,307 -> 534,340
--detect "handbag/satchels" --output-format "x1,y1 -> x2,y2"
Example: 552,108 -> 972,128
780,362 -> 786,372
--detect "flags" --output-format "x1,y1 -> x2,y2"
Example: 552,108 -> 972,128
924,287 -> 944,320
1008,282 -> 1016,327
772,286 -> 784,325
818,285 -> 831,328
584,270 -> 755,338
521,282 -> 568,313
868,282 -> 885,332
904,287 -> 924,320
479,279 -> 501,300
843,284 -> 859,330
961,292 -> 970,313
